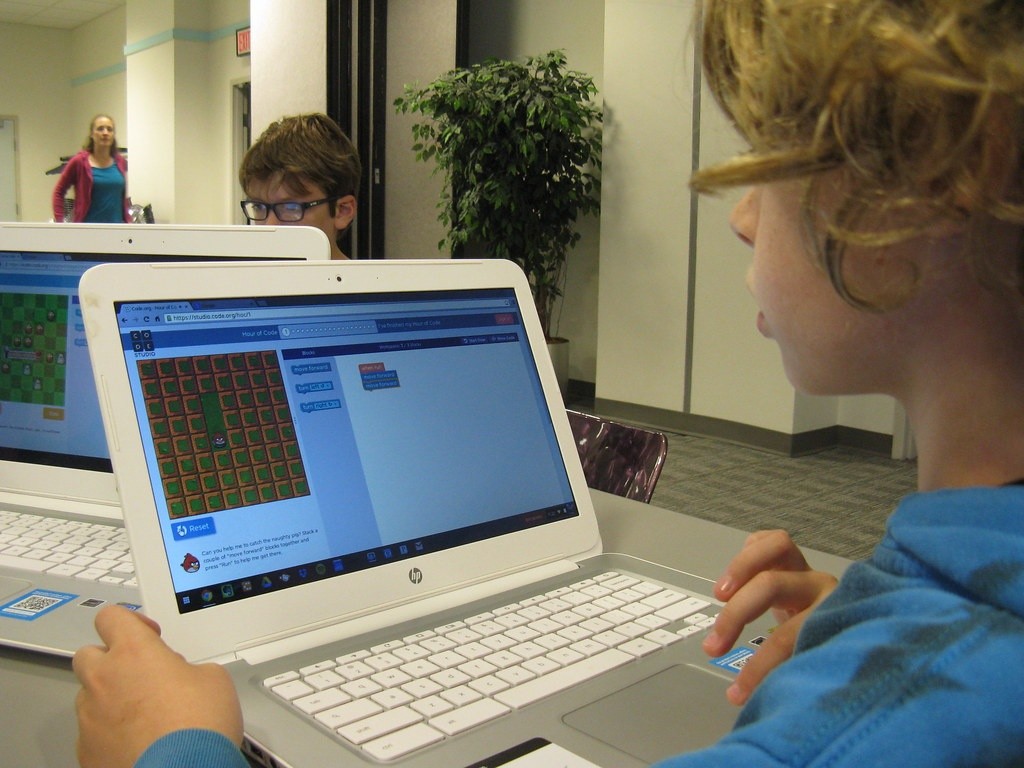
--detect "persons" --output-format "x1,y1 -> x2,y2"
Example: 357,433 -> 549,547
74,1 -> 1024,768
52,114 -> 131,223
238,112 -> 363,260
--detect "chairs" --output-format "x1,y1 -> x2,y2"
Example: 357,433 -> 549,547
567,411 -> 669,505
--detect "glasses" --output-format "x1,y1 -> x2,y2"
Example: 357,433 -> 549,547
241,198 -> 329,222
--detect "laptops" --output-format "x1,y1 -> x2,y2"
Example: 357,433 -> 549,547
0,225 -> 332,657
79,258 -> 789,768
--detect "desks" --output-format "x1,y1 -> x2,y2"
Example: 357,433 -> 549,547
0,487 -> 854,768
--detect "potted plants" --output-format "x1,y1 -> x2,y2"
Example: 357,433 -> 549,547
393,46 -> 603,397
